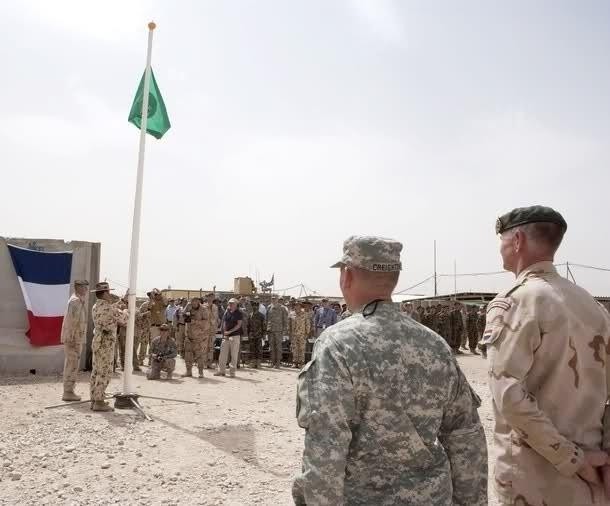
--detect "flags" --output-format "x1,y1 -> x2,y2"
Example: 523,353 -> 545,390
129,67 -> 171,139
5,243 -> 73,347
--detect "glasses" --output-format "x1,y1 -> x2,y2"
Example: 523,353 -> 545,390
160,329 -> 168,331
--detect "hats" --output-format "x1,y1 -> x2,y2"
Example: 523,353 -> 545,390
229,298 -> 239,304
73,279 -> 89,285
90,282 -> 115,293
403,302 -> 414,310
252,296 -> 329,306
495,205 -> 567,234
329,235 -> 402,272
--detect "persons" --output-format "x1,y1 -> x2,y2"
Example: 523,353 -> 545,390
90,283 -> 131,411
285,235 -> 488,506
114,290 -> 351,381
402,301 -> 487,355
478,206 -> 610,506
62,278 -> 90,400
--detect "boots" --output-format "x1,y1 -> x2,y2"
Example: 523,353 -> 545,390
453,346 -> 487,359
248,359 -> 303,369
166,368 -> 235,380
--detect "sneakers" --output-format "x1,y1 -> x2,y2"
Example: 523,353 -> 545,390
91,400 -> 114,411
62,391 -> 80,401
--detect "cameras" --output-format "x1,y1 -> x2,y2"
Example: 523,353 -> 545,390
157,353 -> 164,362
183,312 -> 190,318
